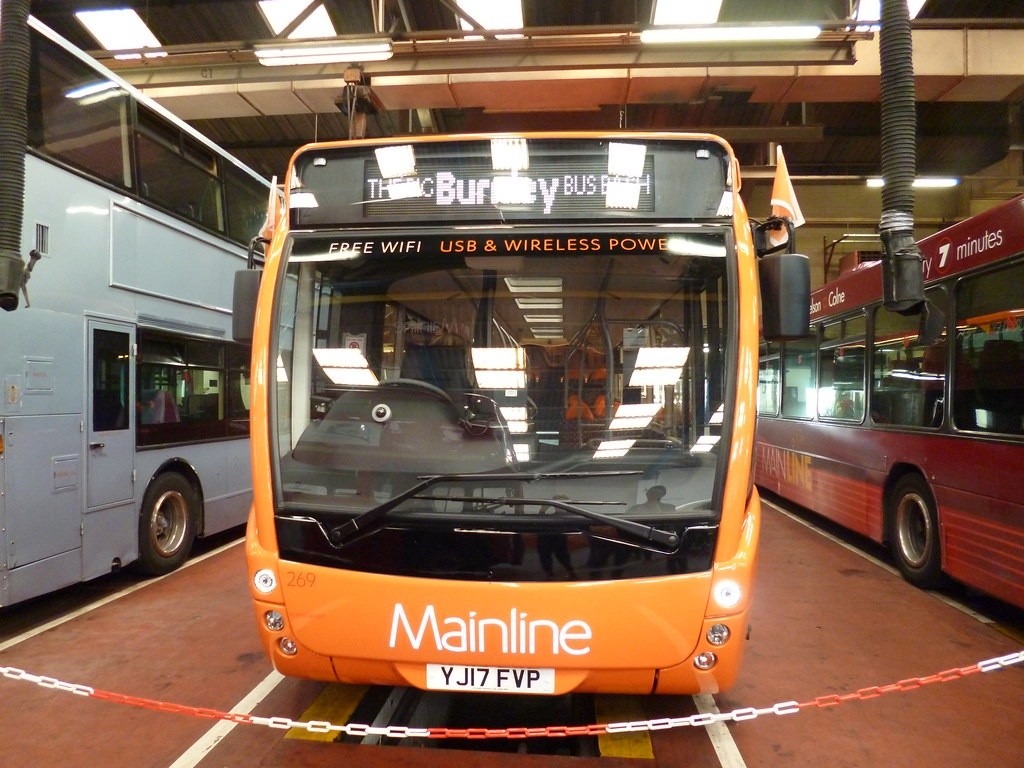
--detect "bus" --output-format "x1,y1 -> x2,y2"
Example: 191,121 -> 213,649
239,124 -> 815,699
0,8 -> 254,611
753,195 -> 1024,622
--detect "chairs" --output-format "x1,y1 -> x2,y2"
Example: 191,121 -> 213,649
529,350 -> 624,421
401,343 -> 474,419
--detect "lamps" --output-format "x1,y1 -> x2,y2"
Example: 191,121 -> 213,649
74,6 -> 171,58
639,23 -> 823,47
253,36 -> 396,68
62,78 -> 130,107
865,177 -> 959,189
